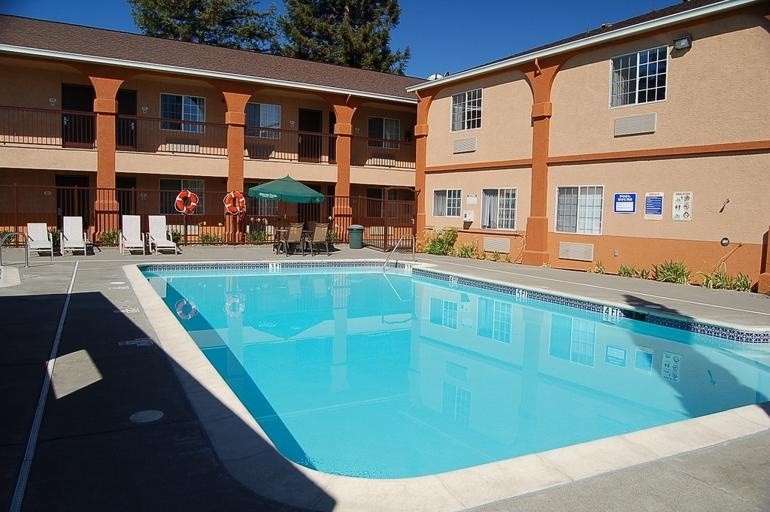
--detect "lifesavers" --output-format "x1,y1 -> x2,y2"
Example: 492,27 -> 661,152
226,297 -> 244,317
177,300 -> 195,320
175,191 -> 198,214
225,193 -> 245,215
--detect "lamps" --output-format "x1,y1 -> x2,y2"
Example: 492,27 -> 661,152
673,33 -> 692,49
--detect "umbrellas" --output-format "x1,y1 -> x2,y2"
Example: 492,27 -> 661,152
241,277 -> 327,344
248,174 -> 326,229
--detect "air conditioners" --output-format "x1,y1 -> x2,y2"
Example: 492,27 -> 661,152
458,302 -> 472,313
463,211 -> 473,223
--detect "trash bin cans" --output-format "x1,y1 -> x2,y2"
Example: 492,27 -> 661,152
347,225 -> 365,249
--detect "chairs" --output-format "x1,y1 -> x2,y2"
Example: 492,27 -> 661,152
24,213 -> 177,261
273,222 -> 330,257
